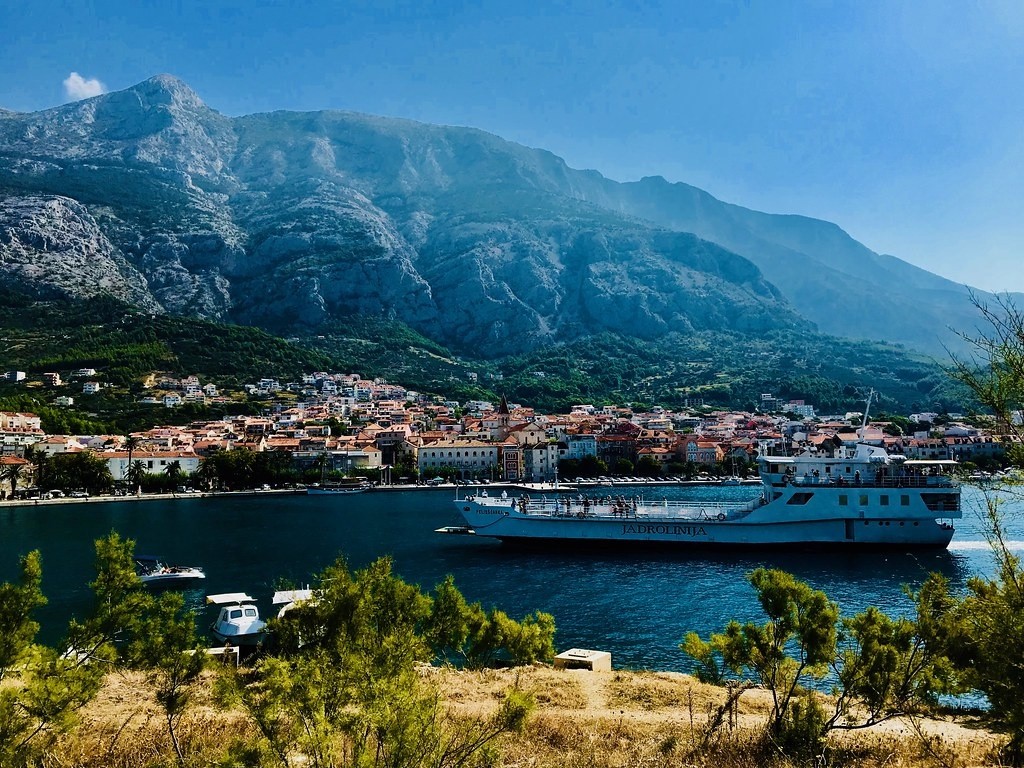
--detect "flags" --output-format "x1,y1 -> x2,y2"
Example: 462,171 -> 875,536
870,391 -> 882,404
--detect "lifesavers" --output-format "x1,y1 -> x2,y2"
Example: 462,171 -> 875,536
718,513 -> 725,522
577,512 -> 585,519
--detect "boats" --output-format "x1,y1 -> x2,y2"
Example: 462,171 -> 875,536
134,558 -> 206,590
451,385 -> 962,552
208,604 -> 269,649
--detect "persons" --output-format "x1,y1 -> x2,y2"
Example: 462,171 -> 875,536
785,466 -> 958,488
463,489 -> 667,519
